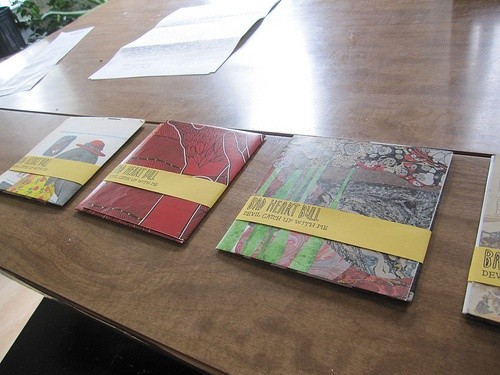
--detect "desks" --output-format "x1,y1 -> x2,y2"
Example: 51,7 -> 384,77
0,0 -> 500,374
